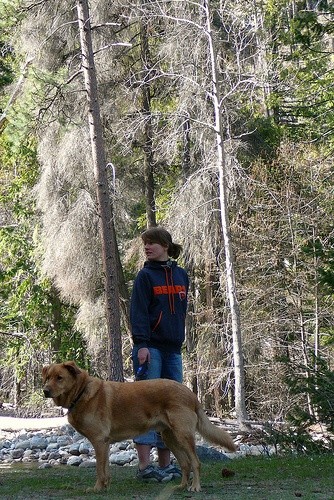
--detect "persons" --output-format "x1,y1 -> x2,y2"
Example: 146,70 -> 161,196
129,228 -> 193,484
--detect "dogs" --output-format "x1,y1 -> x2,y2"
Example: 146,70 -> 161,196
41,360 -> 240,493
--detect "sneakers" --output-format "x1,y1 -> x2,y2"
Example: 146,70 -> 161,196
136,465 -> 173,483
159,464 -> 182,478
